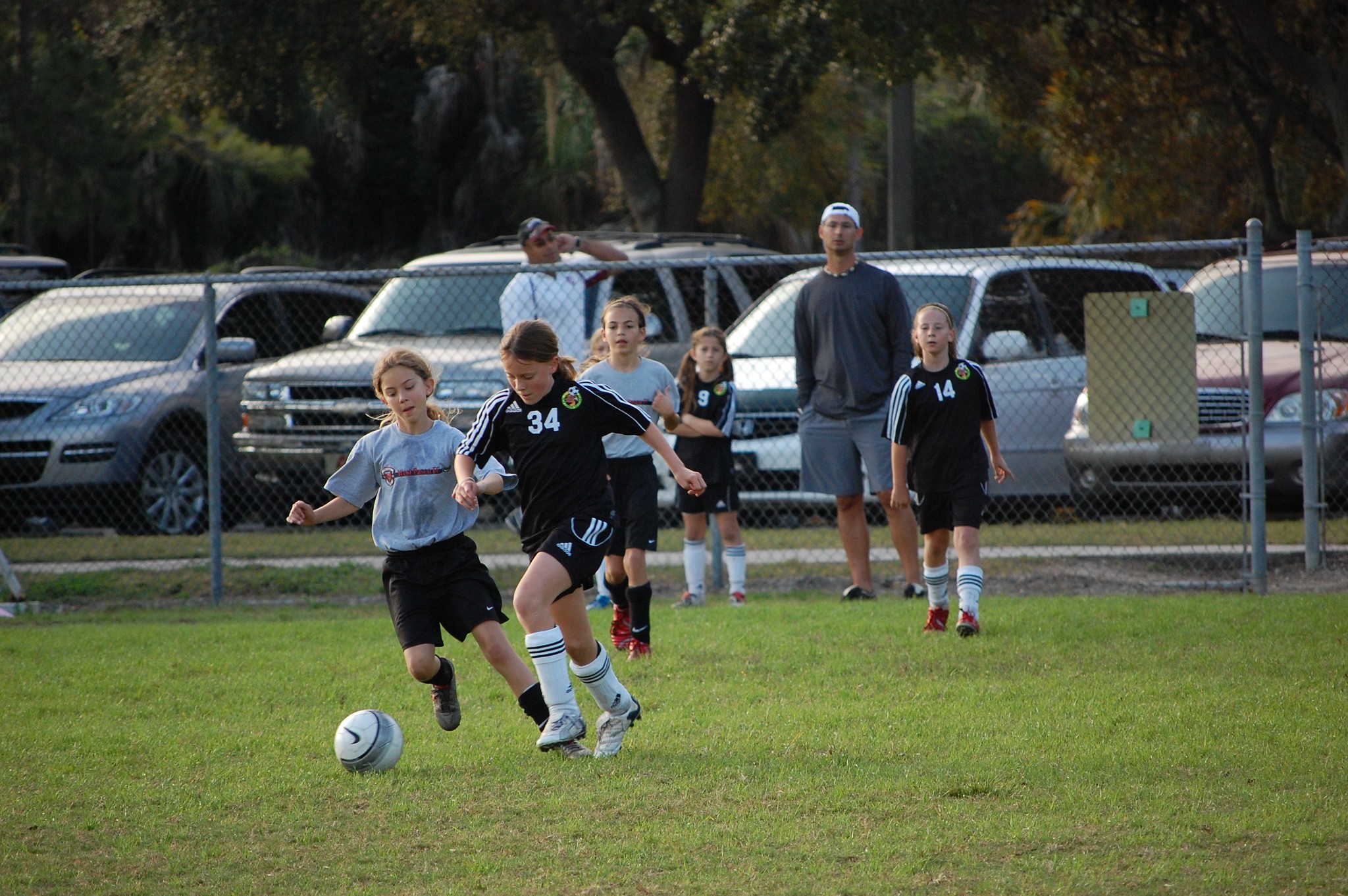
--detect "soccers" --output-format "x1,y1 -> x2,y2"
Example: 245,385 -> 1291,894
331,709 -> 404,776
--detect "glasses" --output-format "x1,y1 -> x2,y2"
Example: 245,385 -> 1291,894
528,233 -> 554,248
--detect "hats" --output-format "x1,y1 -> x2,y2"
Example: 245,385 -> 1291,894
518,217 -> 555,248
820,203 -> 860,228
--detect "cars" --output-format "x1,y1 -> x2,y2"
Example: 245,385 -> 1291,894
652,252 -> 1177,523
1062,250 -> 1348,518
0,266 -> 379,538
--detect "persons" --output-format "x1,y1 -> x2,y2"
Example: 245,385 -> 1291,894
500,218 -> 629,544
287,297 -> 746,758
882,303 -> 1012,635
790,204 -> 928,603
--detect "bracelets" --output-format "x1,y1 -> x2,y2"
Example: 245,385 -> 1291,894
573,236 -> 581,251
458,477 -> 475,487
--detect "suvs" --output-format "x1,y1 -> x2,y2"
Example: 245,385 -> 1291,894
232,225 -> 788,524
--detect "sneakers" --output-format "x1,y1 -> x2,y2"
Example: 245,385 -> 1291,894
905,582 -> 926,599
729,591 -> 745,606
923,607 -> 948,634
672,591 -> 704,608
839,585 -> 876,601
585,595 -> 612,610
431,658 -> 461,731
628,638 -> 650,661
956,608 -> 980,636
560,739 -> 593,759
592,696 -> 642,758
610,603 -> 631,650
536,711 -> 588,749
